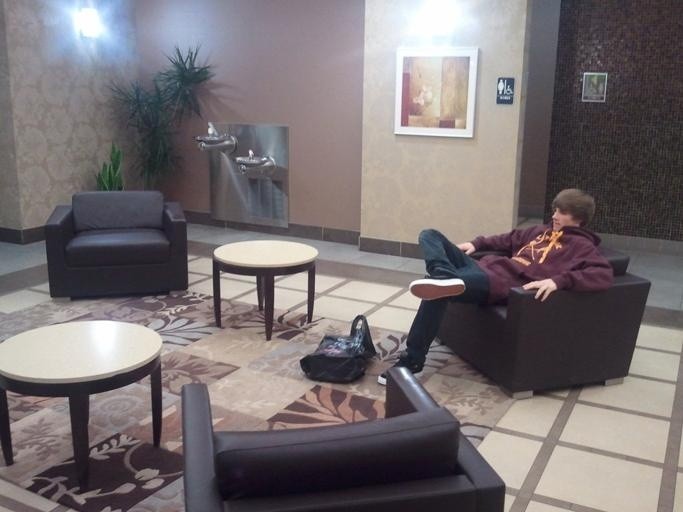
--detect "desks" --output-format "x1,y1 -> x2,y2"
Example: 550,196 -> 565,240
0,321 -> 163,480
212,240 -> 318,341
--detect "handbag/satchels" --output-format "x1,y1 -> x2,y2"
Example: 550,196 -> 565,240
300,315 -> 376,384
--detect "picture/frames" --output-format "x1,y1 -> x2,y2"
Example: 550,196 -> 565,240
394,48 -> 478,139
582,74 -> 608,102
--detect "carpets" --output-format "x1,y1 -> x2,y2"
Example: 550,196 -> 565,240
0,288 -> 525,512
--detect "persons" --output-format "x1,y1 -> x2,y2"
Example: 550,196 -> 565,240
377,188 -> 614,387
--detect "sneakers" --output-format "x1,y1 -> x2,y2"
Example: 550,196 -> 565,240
378,352 -> 425,385
409,268 -> 465,300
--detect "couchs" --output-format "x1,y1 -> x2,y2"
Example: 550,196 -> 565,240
184,368 -> 505,512
46,189 -> 189,301
436,243 -> 650,400
